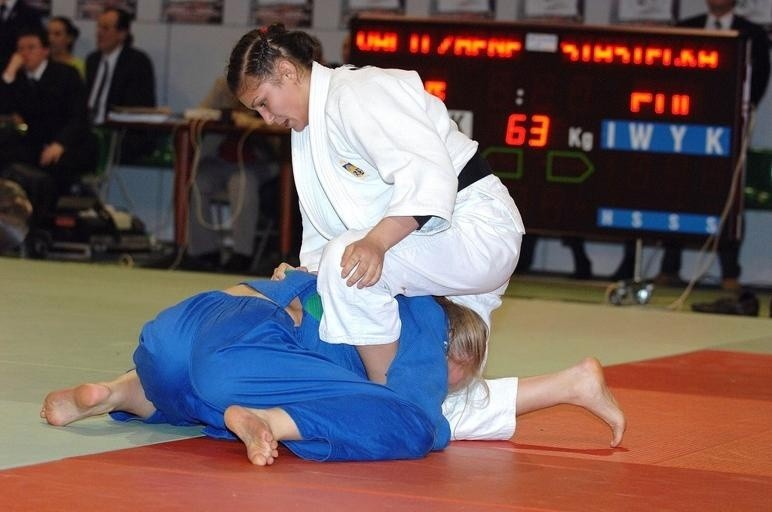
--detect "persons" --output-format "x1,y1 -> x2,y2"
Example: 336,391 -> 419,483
0,0 -> 157,253
650,0 -> 772,293
41,266 -> 490,468
184,71 -> 281,268
223,21 -> 627,448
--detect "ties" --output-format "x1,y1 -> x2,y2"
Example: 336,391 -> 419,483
91,60 -> 107,119
